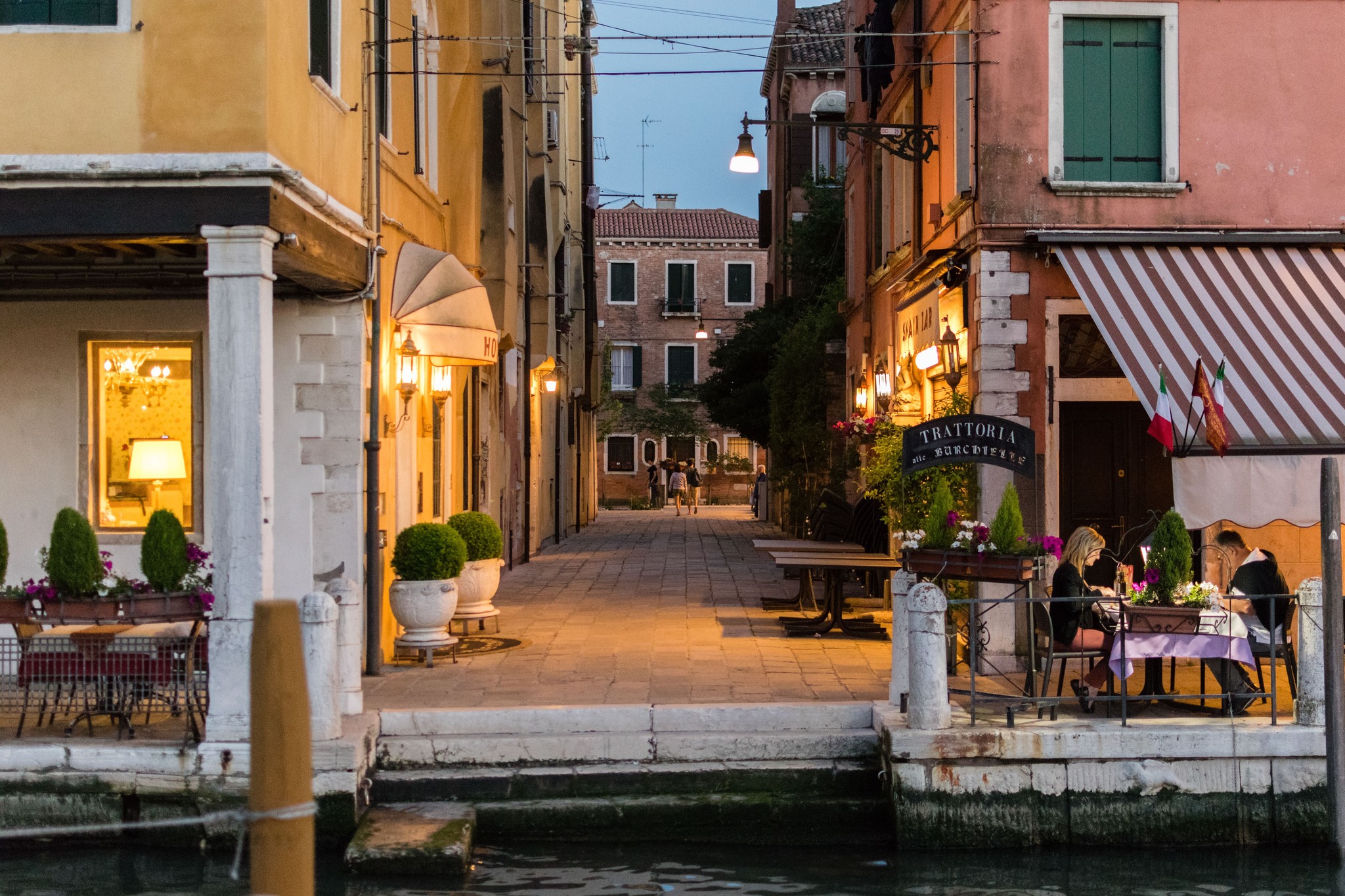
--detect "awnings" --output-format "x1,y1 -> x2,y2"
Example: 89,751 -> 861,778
1053,243 -> 1345,530
389,242 -> 498,366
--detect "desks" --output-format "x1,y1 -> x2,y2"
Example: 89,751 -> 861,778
752,538 -> 901,640
29,621 -> 193,740
1109,608 -> 1257,715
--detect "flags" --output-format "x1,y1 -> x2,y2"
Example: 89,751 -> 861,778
1191,358 -> 1230,458
1147,369 -> 1173,452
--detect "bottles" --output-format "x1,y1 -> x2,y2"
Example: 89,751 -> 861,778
1113,571 -> 1126,596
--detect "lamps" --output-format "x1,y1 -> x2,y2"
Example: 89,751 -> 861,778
728,111 -> 939,173
696,316 -> 754,339
384,329 -> 452,438
105,347 -> 187,511
534,369 -> 557,391
857,315 -> 963,416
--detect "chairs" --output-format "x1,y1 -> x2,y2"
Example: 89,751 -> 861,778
1019,600 -> 1298,720
11,619 -> 204,743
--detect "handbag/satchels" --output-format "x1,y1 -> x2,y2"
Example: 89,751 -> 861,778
693,468 -> 702,487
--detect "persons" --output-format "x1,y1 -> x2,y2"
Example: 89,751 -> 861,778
683,458 -> 702,515
751,465 -> 766,519
646,458 -> 658,506
1049,526 -> 1115,713
668,464 -> 687,515
1200,529 -> 1290,714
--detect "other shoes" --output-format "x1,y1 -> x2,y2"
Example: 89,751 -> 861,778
751,516 -> 758,519
1071,680 -> 1082,704
694,507 -> 697,514
1077,687 -> 1095,714
689,511 -> 691,515
1247,683 -> 1261,708
1229,687 -> 1256,714
677,510 -> 680,515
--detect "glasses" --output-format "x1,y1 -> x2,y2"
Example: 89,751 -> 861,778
1217,545 -> 1235,561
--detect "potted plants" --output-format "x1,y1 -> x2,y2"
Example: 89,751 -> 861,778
559,307 -> 576,334
388,511 -> 506,641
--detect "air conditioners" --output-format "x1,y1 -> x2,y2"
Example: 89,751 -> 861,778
546,109 -> 558,148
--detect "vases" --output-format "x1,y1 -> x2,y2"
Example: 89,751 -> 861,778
1123,601 -> 1202,633
904,549 -> 1037,580
0,591 -> 203,623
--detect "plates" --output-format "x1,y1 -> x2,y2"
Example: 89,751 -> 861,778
1109,604 -> 1119,610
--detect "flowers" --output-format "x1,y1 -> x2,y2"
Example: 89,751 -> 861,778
834,415 -> 880,439
893,464 -> 1064,561
0,506 -> 216,608
1129,509 -> 1224,612
